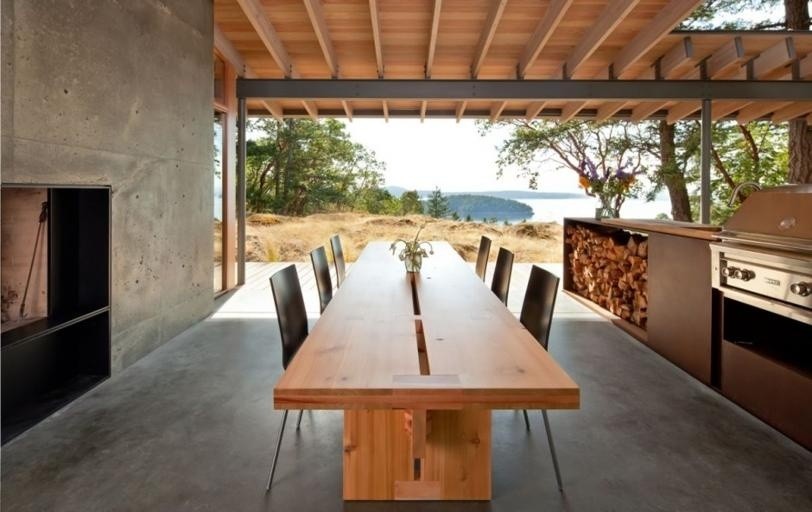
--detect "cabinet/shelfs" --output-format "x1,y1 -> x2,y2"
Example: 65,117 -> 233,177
0,183 -> 110,444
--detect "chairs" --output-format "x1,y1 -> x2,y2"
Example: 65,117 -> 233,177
519,265 -> 560,351
309,246 -> 332,314
491,247 -> 514,307
270,264 -> 308,370
476,237 -> 491,281
330,236 -> 346,286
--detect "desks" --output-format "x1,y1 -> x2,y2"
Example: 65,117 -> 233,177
275,242 -> 579,502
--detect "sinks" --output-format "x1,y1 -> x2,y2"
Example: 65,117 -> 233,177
681,226 -> 723,234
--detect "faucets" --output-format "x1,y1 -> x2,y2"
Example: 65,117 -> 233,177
727,181 -> 760,207
